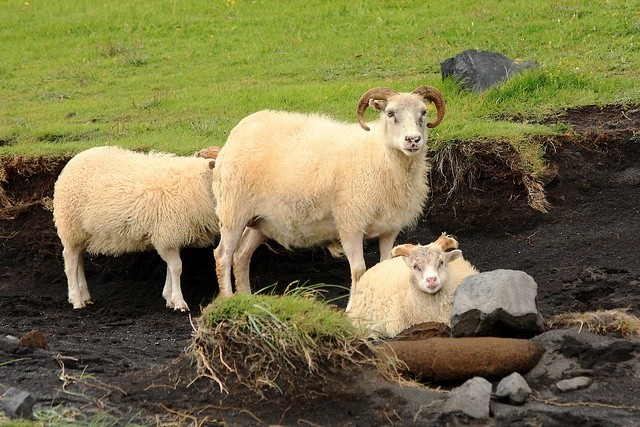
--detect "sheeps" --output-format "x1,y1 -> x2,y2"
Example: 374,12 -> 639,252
349,232 -> 479,339
211,85 -> 446,313
53,146 -> 221,313
